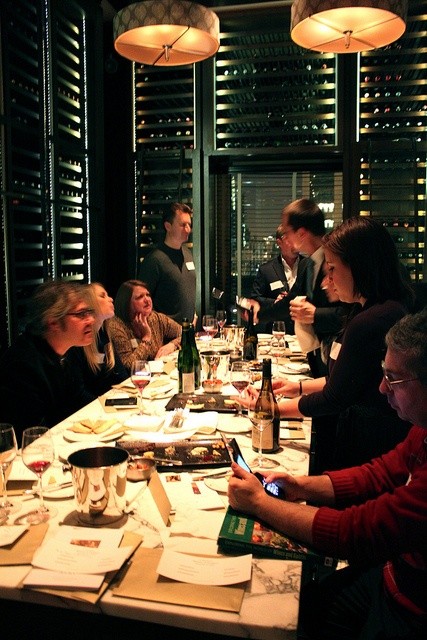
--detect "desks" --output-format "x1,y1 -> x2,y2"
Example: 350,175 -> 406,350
0,334 -> 312,639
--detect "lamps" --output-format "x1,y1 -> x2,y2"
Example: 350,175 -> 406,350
114,0 -> 219,66
292,0 -> 410,55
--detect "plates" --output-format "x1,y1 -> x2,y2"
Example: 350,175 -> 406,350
165,394 -> 249,412
204,467 -> 233,492
116,438 -> 244,472
138,379 -> 178,399
32,468 -> 74,498
217,417 -> 253,434
63,417 -> 124,441
279,362 -> 310,374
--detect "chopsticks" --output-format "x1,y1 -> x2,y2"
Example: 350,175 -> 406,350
193,470 -> 233,481
23,481 -> 73,495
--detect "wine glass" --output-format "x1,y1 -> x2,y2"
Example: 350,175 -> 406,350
0,423 -> 18,508
206,319 -> 218,351
130,361 -> 151,418
21,427 -> 55,521
203,315 -> 214,350
230,361 -> 252,418
216,311 -> 227,342
247,392 -> 280,471
272,321 -> 285,351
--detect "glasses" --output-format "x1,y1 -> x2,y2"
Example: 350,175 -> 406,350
380,361 -> 420,390
279,229 -> 293,239
62,307 -> 95,318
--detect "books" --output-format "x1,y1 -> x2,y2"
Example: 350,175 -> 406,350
216,506 -> 338,569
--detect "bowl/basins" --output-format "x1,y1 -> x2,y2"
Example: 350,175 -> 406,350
127,458 -> 156,481
257,334 -> 273,345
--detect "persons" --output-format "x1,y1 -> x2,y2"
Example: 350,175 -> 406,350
66,283 -> 130,396
243,198 -> 347,332
142,202 -> 195,321
104,280 -> 182,369
321,259 -> 340,303
235,216 -> 415,468
0,281 -> 96,433
228,313 -> 427,639
252,222 -> 306,332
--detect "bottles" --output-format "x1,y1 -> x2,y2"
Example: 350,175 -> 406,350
188,323 -> 201,390
252,359 -> 280,454
178,318 -> 195,395
243,305 -> 258,361
230,294 -> 241,326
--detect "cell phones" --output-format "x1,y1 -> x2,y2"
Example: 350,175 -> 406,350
231,451 -> 286,499
104,396 -> 140,409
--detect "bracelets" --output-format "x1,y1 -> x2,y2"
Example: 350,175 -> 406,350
299,380 -> 304,397
140,339 -> 152,345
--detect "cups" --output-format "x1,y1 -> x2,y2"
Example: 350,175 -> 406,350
59,446 -> 138,526
